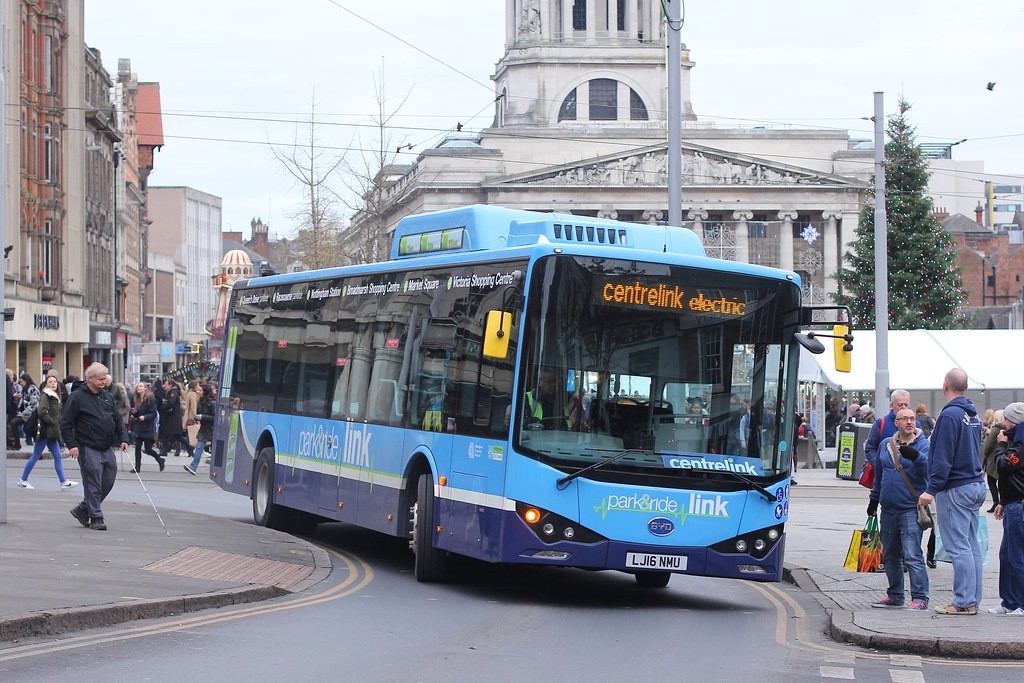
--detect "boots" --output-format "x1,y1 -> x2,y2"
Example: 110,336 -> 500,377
792,455 -> 797,473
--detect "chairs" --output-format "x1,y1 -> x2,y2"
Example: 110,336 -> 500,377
236,322 -> 679,458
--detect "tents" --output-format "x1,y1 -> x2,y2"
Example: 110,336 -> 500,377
746,329 -> 1024,469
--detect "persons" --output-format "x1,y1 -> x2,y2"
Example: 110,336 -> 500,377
17,375 -> 79,489
591,389 -> 612,400
686,391 -> 822,486
504,364 -> 584,432
620,389 -> 627,396
825,393 -> 875,446
864,367 -> 1024,615
563,149 -> 790,188
634,391 -> 640,397
59,362 -> 130,530
6,368 -> 218,475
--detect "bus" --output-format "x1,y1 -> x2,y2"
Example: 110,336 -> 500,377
210,204 -> 855,590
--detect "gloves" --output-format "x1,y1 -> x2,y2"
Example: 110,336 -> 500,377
866,498 -> 879,517
898,445 -> 919,461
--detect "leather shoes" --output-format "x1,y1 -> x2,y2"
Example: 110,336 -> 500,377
926,556 -> 937,568
903,563 -> 908,572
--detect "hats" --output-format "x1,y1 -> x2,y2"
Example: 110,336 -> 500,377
1004,402 -> 1024,426
860,405 -> 870,414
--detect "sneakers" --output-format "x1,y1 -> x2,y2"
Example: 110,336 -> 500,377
988,604 -> 1015,614
17,479 -> 35,490
71,505 -> 90,528
61,479 -> 79,490
1006,607 -> 1024,617
935,602 -> 978,614
870,597 -> 905,609
905,602 -> 929,611
90,516 -> 107,532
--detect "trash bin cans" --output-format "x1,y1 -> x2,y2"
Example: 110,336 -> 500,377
836,422 -> 873,481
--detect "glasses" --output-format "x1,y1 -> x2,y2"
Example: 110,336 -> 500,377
896,416 -> 916,422
539,375 -> 560,385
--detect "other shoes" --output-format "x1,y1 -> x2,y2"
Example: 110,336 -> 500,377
987,504 -> 998,513
6,431 -> 212,476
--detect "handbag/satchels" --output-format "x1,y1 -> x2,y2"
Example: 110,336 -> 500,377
859,458 -> 875,489
22,411 -> 47,441
933,509 -> 991,565
842,514 -> 885,572
916,502 -> 933,531
